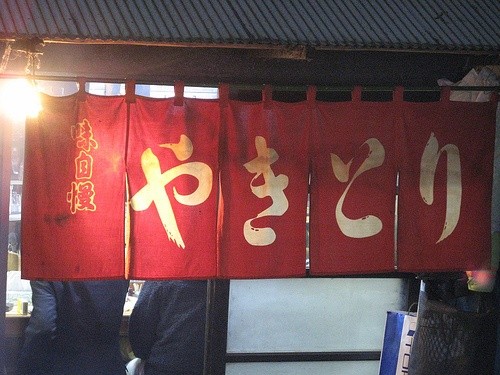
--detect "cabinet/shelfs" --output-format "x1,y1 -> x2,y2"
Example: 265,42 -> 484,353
7,139 -> 26,270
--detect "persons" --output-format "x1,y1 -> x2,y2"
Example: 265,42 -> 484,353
15,278 -> 128,375
130,278 -> 208,374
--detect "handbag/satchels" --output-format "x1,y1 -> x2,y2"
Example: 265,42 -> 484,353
378,302 -> 418,375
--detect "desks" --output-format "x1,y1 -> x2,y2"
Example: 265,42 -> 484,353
4,271 -> 139,337
